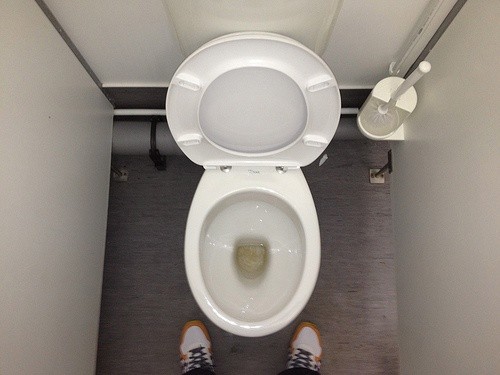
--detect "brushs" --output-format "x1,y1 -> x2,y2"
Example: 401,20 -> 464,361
360,60 -> 432,136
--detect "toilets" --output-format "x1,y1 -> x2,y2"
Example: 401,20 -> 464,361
165,30 -> 341,339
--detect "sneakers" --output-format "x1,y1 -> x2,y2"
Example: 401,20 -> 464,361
286,320 -> 323,373
176,319 -> 215,374
